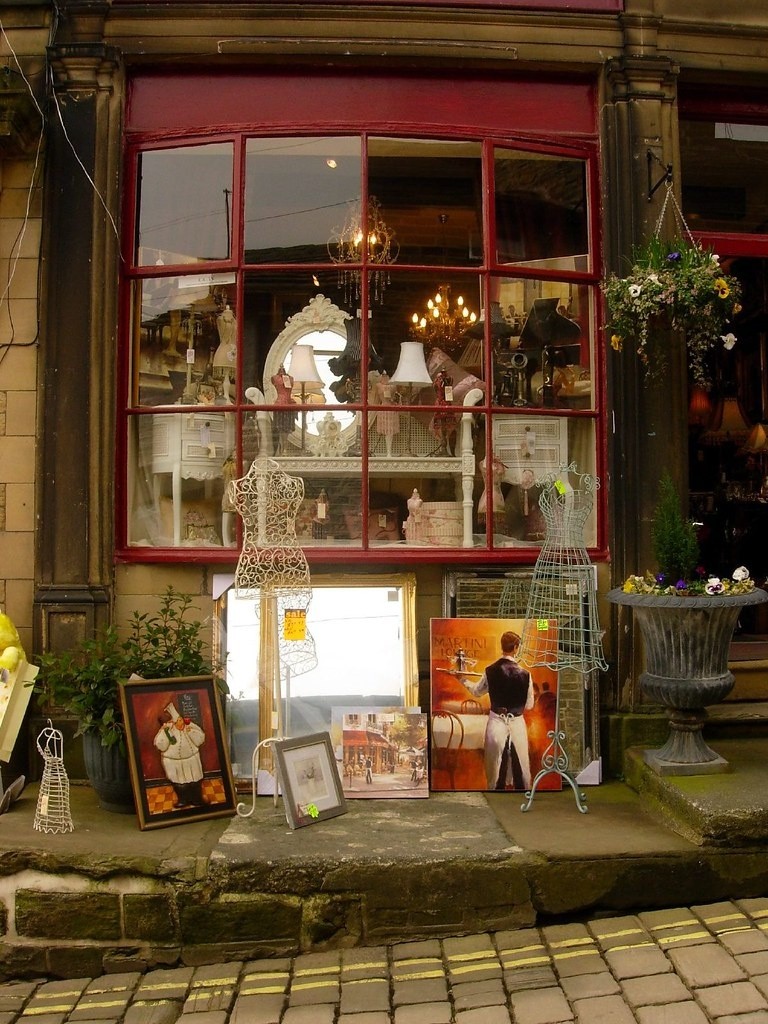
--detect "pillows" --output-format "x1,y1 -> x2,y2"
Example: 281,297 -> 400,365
159,495 -> 223,546
411,347 -> 485,441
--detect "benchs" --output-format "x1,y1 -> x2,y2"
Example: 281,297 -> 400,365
245,387 -> 483,547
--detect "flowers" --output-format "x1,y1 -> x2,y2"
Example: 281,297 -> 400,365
597,226 -> 742,390
624,475 -> 754,596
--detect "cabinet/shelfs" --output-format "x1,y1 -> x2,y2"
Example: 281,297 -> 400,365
151,405 -> 234,546
482,413 -> 574,525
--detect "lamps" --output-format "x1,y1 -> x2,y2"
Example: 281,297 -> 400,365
288,346 -> 325,450
733,423 -> 768,474
387,342 -> 433,456
409,215 -> 476,353
700,396 -> 751,444
688,391 -> 712,427
291,389 -> 326,404
327,196 -> 400,307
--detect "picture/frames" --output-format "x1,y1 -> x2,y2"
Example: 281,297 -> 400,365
212,574 -> 419,792
442,564 -> 602,786
117,675 -> 237,831
272,731 -> 348,830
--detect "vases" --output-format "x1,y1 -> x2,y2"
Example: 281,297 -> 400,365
604,586 -> 768,775
650,316 -> 671,330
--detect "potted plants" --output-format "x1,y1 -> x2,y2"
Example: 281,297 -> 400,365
22,585 -> 233,814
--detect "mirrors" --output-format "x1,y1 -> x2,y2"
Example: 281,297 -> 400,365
263,295 -> 381,457
